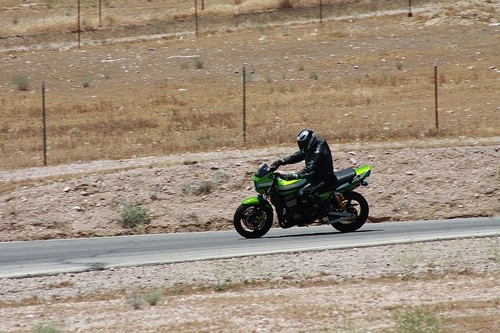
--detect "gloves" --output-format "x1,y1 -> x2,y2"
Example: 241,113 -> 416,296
280,173 -> 296,181
270,158 -> 286,171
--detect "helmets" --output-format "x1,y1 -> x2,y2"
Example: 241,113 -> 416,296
296,129 -> 318,153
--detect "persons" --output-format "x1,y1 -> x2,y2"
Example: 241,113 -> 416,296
269,129 -> 335,219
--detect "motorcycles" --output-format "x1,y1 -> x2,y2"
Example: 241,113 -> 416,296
233,162 -> 371,238
